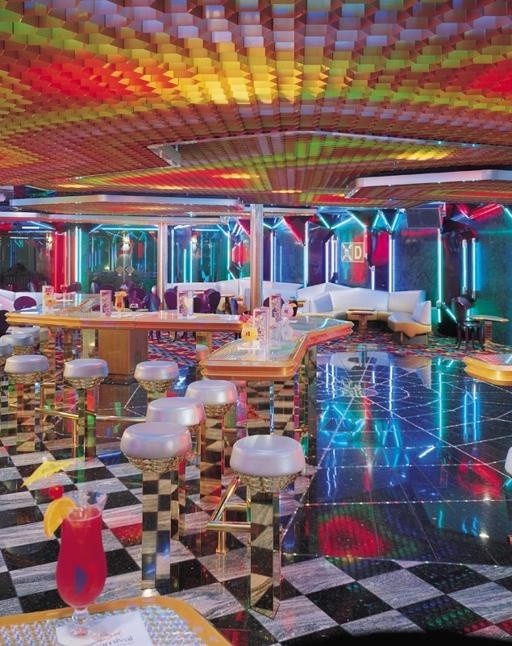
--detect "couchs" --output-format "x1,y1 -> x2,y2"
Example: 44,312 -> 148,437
151,276 -> 250,312
264,282 -> 432,346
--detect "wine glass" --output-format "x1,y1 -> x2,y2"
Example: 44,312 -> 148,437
114,291 -> 124,316
55,489 -> 108,645
241,317 -> 259,355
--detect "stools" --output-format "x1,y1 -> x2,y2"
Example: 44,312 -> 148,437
185,379 -> 237,505
505,447 -> 512,545
147,397 -> 204,541
230,434 -> 306,619
120,422 -> 192,589
0,326 -> 179,462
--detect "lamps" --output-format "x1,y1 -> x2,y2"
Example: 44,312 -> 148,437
147,142 -> 182,169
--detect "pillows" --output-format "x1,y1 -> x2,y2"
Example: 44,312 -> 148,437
313,293 -> 333,313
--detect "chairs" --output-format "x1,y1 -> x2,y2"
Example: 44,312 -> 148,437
98,285 -> 114,301
454,300 -> 484,353
208,291 -> 221,314
231,298 -> 241,340
14,296 -> 37,328
129,284 -> 178,342
206,289 -> 215,305
225,296 -> 231,314
194,297 -> 202,313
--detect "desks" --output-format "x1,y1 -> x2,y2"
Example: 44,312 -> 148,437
4,293 -> 354,381
0,597 -> 232,646
471,315 -> 509,352
465,353 -> 511,387
